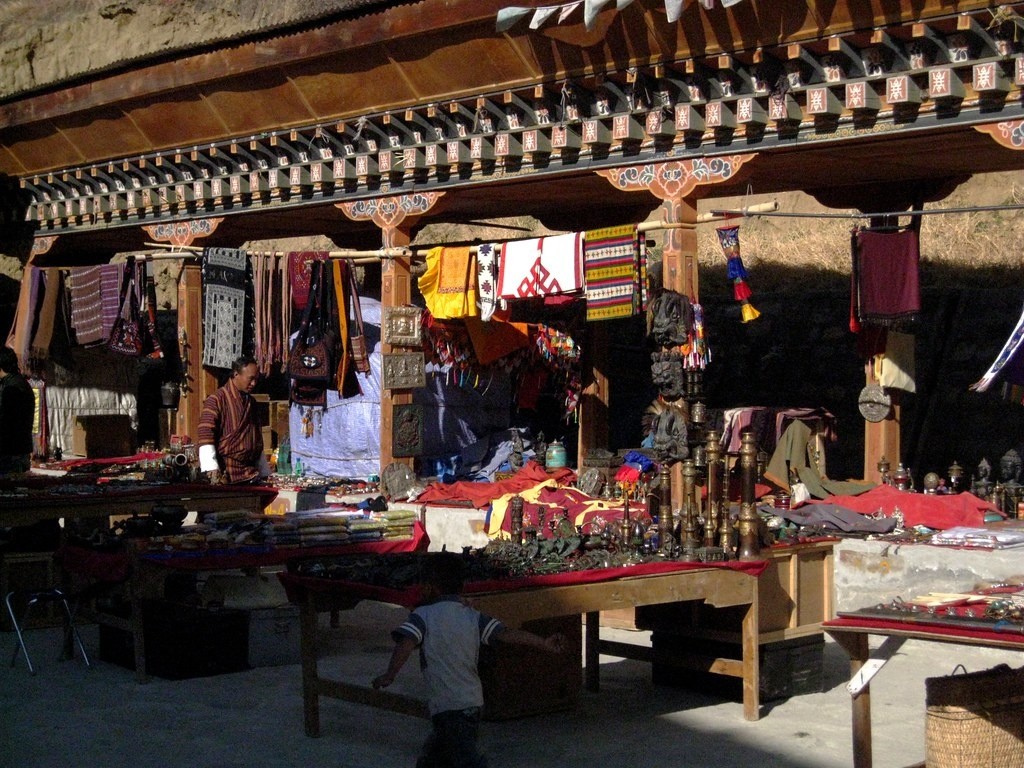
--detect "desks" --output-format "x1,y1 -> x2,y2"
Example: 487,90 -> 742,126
820,585 -> 1024,768
275,558 -> 770,738
0,483 -> 280,528
62,505 -> 430,684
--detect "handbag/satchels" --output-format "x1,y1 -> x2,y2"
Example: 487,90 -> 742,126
287,324 -> 339,385
350,334 -> 369,372
236,444 -> 263,466
106,318 -> 143,357
288,379 -> 328,417
336,352 -> 365,400
141,322 -> 166,360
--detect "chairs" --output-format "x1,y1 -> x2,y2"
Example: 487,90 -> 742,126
0,523 -> 91,676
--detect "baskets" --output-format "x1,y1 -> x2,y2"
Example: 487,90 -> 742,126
923,663 -> 1024,768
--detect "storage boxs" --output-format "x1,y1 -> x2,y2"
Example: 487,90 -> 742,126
649,635 -> 851,702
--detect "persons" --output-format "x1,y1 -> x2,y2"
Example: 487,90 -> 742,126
0,345 -> 35,475
974,449 -> 1024,516
198,356 -> 271,485
370,552 -> 565,767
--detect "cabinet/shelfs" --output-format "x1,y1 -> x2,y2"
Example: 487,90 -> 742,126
635,539 -> 844,646
